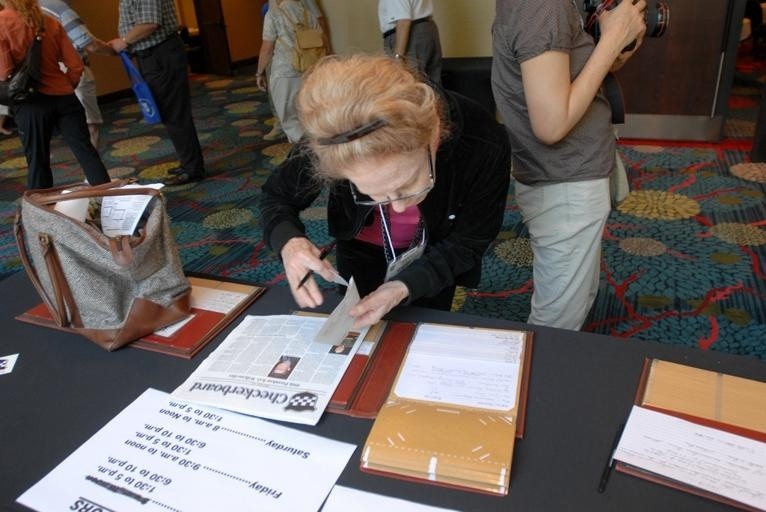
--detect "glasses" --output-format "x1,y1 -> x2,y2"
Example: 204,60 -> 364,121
350,145 -> 435,205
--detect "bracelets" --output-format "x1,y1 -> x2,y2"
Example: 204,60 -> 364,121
122,36 -> 130,46
393,52 -> 404,59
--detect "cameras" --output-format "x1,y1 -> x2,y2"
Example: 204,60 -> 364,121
584,0 -> 670,54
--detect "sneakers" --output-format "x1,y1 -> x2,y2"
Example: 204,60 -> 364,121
263,129 -> 285,141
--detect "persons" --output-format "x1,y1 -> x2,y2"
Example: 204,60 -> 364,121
377,1 -> 443,82
334,336 -> 355,353
35,1 -> 115,148
106,1 -> 206,187
0,1 -> 111,187
490,0 -> 647,334
252,0 -> 333,144
259,55 -> 512,329
272,356 -> 297,379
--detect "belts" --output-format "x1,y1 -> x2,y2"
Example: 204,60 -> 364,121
383,16 -> 434,39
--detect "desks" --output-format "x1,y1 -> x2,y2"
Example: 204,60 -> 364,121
3,270 -> 763,512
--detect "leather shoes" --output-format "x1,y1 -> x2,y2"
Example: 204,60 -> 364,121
168,166 -> 184,175
164,173 -> 189,185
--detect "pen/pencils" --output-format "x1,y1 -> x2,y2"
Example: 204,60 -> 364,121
599,422 -> 626,492
295,239 -> 338,290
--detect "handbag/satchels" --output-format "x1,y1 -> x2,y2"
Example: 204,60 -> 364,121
0,34 -> 43,106
132,81 -> 162,125
13,178 -> 192,353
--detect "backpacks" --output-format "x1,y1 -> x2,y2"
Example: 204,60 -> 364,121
274,7 -> 327,73
